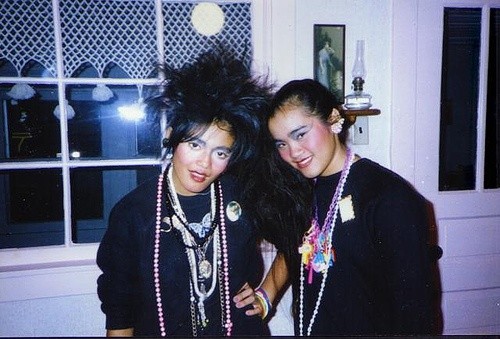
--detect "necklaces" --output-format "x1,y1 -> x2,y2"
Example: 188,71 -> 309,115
154,160 -> 234,337
296,144 -> 356,337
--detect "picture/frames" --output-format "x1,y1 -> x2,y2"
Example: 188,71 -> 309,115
314,24 -> 346,104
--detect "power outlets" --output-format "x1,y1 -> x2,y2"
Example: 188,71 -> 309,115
354,116 -> 369,146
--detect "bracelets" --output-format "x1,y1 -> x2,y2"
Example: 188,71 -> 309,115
254,289 -> 272,320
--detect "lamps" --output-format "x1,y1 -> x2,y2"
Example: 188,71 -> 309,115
345,39 -> 372,111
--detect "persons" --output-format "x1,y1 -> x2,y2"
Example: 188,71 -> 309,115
96,59 -> 294,337
266,79 -> 444,339
